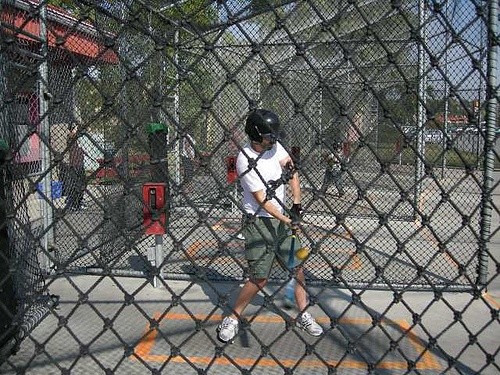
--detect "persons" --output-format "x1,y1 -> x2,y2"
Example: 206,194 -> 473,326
180,127 -> 197,183
216,108 -> 324,343
320,141 -> 348,199
64,121 -> 96,211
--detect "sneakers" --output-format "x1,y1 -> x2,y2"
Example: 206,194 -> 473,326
219,317 -> 239,342
296,312 -> 324,337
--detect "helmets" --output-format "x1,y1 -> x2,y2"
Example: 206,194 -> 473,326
245,109 -> 281,151
68,120 -> 80,132
333,141 -> 343,153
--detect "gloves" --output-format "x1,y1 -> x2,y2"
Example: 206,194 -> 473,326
289,204 -> 302,225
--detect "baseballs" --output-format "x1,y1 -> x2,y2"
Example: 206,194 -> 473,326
296,248 -> 308,259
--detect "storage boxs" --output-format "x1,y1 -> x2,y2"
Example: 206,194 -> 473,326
37,181 -> 64,200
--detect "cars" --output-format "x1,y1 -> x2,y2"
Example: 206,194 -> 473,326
426,129 -> 444,143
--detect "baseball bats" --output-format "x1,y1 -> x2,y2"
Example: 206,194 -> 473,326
285,229 -> 296,308
